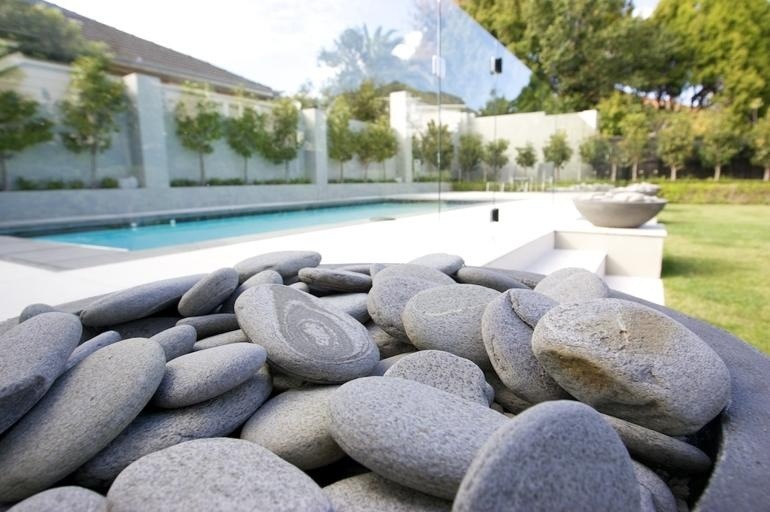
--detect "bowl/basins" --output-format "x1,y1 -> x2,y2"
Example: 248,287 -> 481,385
571,196 -> 666,228
